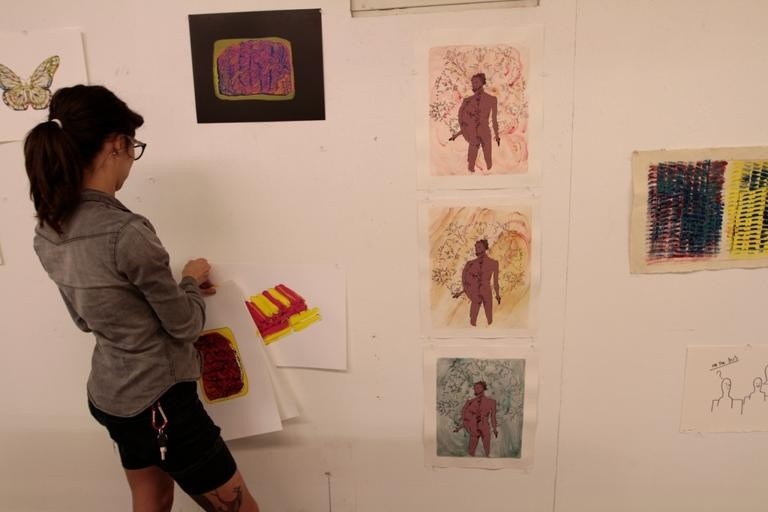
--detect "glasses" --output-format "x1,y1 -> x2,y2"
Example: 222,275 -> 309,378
109,131 -> 147,163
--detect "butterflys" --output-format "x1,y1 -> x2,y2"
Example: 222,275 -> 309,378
0,55 -> 60,111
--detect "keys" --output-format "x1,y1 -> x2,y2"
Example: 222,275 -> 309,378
155,434 -> 168,461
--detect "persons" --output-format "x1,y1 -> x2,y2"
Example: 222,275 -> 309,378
21,84 -> 261,511
449,71 -> 502,172
452,234 -> 502,331
453,381 -> 500,458
